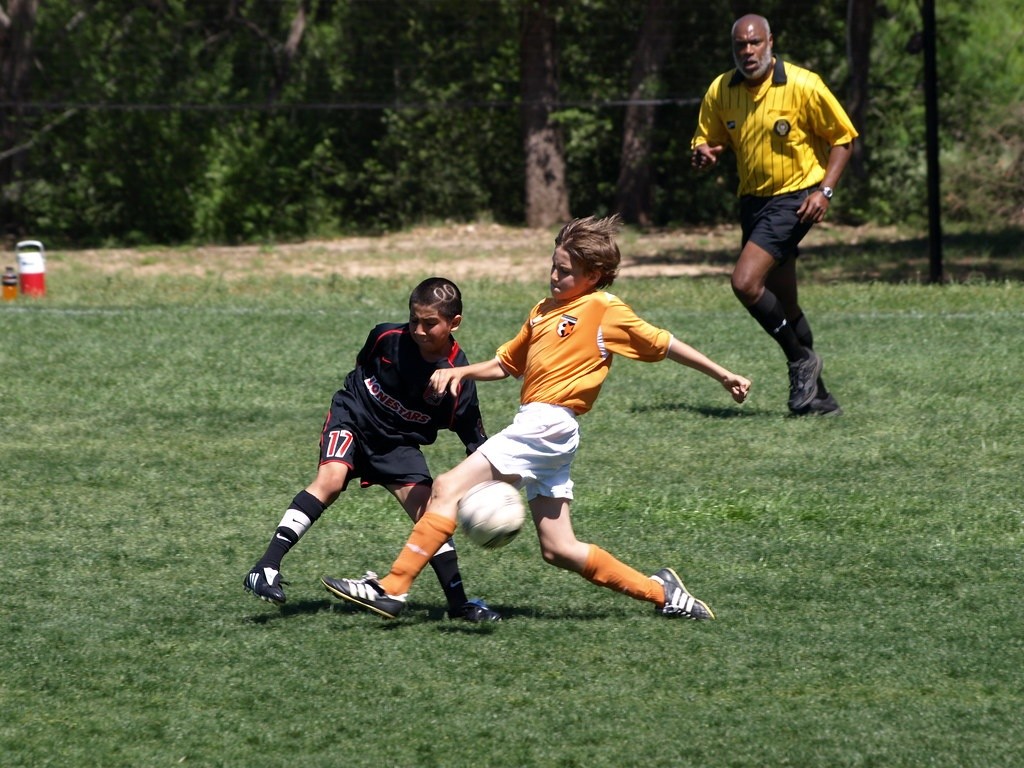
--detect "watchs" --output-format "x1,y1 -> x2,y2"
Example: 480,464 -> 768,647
816,186 -> 834,201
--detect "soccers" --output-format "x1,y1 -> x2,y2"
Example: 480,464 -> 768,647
456,480 -> 527,549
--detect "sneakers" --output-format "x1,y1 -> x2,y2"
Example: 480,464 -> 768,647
787,347 -> 823,410
243,565 -> 291,606
321,571 -> 407,620
442,599 -> 503,623
788,393 -> 841,419
650,568 -> 715,620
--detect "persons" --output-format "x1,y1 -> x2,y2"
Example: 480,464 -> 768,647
321,213 -> 752,623
241,277 -> 503,622
690,14 -> 859,416
